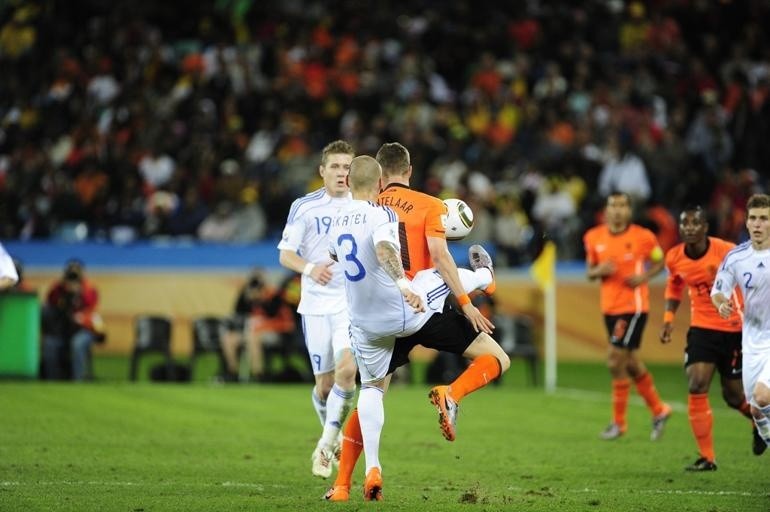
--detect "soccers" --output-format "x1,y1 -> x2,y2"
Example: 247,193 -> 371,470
443,198 -> 474,238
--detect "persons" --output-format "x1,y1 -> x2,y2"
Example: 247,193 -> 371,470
327,155 -> 495,499
583,193 -> 670,442
1,2 -> 769,384
661,202 -> 766,471
320,143 -> 512,500
279,136 -> 356,481
709,194 -> 769,446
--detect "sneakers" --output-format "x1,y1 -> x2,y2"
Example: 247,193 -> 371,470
649,403 -> 671,441
311,445 -> 333,480
333,442 -> 343,469
468,244 -> 496,296
598,423 -> 627,440
752,419 -> 768,455
320,483 -> 350,502
429,384 -> 459,441
684,457 -> 720,474
361,466 -> 385,502
